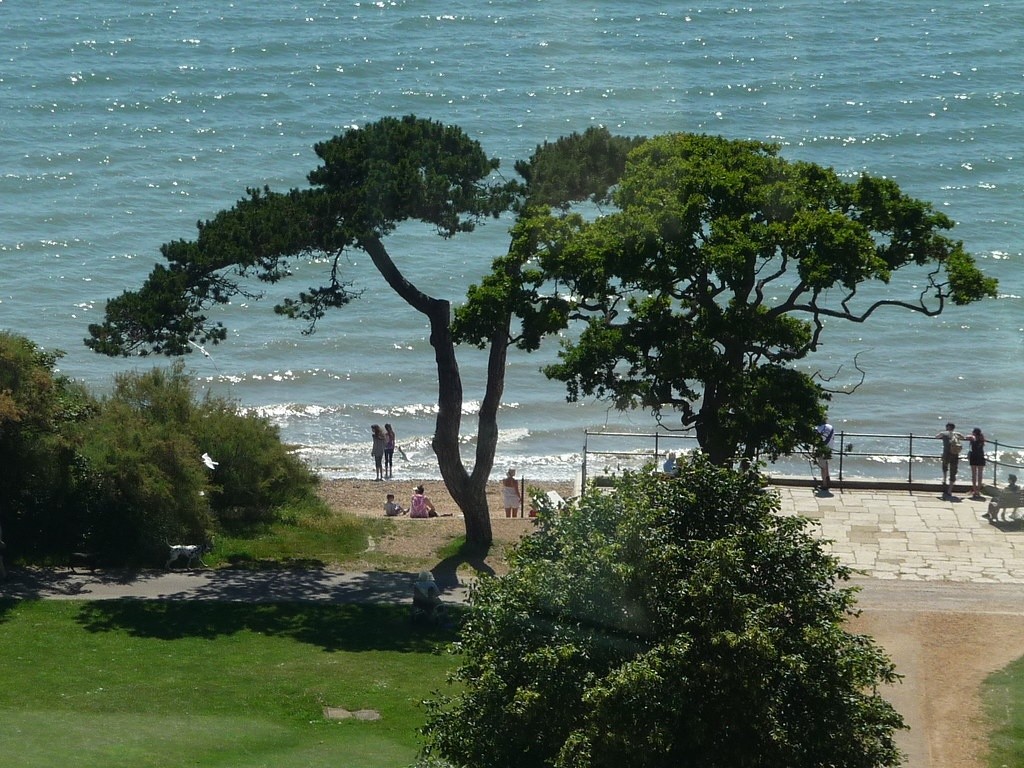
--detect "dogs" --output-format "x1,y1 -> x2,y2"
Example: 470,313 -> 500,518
165,537 -> 210,571
68,550 -> 103,575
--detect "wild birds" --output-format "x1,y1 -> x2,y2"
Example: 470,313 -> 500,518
202,453 -> 219,469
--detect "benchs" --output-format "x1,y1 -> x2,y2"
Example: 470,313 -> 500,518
988,489 -> 1024,524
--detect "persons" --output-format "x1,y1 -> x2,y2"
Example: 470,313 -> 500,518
409,484 -> 439,518
382,422 -> 396,479
980,474 -> 1021,518
369,424 -> 386,482
966,426 -> 986,498
815,413 -> 834,490
500,467 -> 521,518
934,422 -> 964,499
381,492 -> 410,517
663,451 -> 677,475
